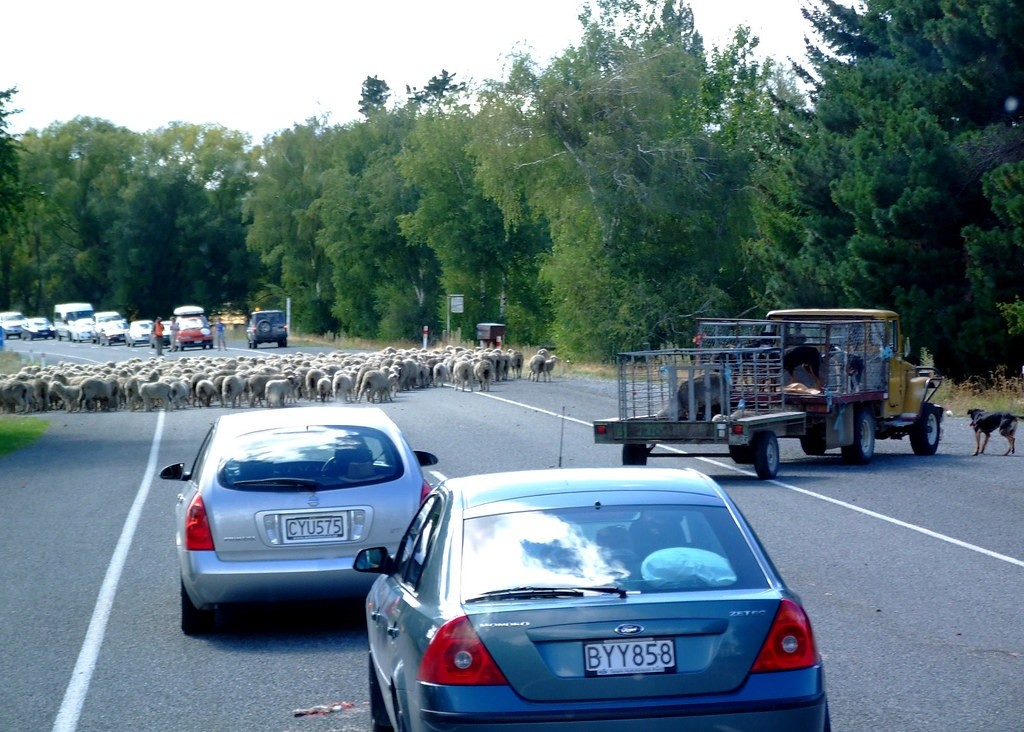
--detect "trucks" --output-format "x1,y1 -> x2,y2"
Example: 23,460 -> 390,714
592,307 -> 946,480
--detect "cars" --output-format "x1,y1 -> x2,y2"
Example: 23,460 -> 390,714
21,318 -> 56,340
152,321 -> 175,348
158,402 -> 440,634
125,320 -> 154,347
101,320 -> 129,345
350,466 -> 830,732
71,318 -> 97,342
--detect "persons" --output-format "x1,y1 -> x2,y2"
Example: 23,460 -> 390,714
167,317 -> 179,353
153,317 -> 164,356
215,318 -> 227,351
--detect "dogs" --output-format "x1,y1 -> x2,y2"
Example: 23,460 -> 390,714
967,408 -> 1024,457
656,373 -> 737,420
710,344 -> 865,392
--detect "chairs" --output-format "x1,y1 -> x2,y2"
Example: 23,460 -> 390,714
611,517 -> 686,563
320,434 -> 375,482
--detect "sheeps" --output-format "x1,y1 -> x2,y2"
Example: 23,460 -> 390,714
0,345 -> 562,416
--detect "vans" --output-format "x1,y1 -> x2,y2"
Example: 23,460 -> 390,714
53,302 -> 93,341
88,311 -> 122,343
0,312 -> 24,338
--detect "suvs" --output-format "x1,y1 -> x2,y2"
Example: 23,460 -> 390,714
171,305 -> 213,351
246,311 -> 287,348
